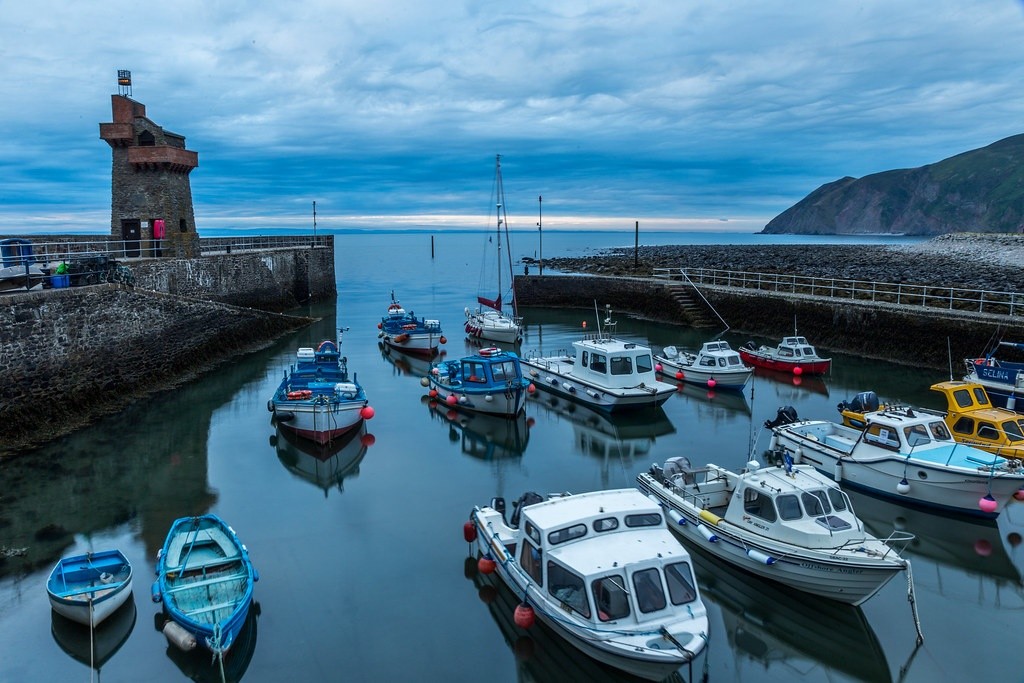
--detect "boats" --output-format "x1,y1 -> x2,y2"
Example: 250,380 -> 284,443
655,371 -> 753,419
520,298 -> 679,415
467,487 -> 709,681
634,447 -> 914,606
962,340 -> 1024,416
267,326 -> 375,443
667,525 -> 897,682
525,379 -> 677,486
737,313 -> 831,377
273,419 -> 367,496
162,608 -> 258,683
152,514 -> 261,666
381,341 -> 443,379
837,337 -> 1024,459
763,391 -> 1024,526
749,366 -> 831,400
427,396 -> 533,461
653,337 -> 756,391
417,343 -> 530,418
51,593 -> 137,683
377,288 -> 443,354
837,487 -> 1023,612
45,549 -> 134,628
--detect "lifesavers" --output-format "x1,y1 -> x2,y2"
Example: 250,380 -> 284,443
479,347 -> 502,356
287,390 -> 312,400
317,340 -> 338,352
403,324 -> 418,329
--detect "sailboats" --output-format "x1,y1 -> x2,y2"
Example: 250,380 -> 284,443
466,333 -> 521,358
464,153 -> 523,344
